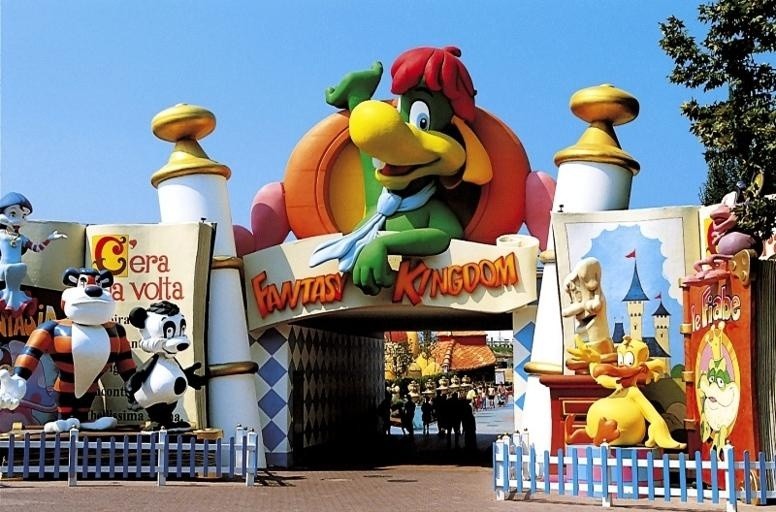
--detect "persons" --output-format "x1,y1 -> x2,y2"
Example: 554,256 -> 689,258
374,379 -> 508,454
